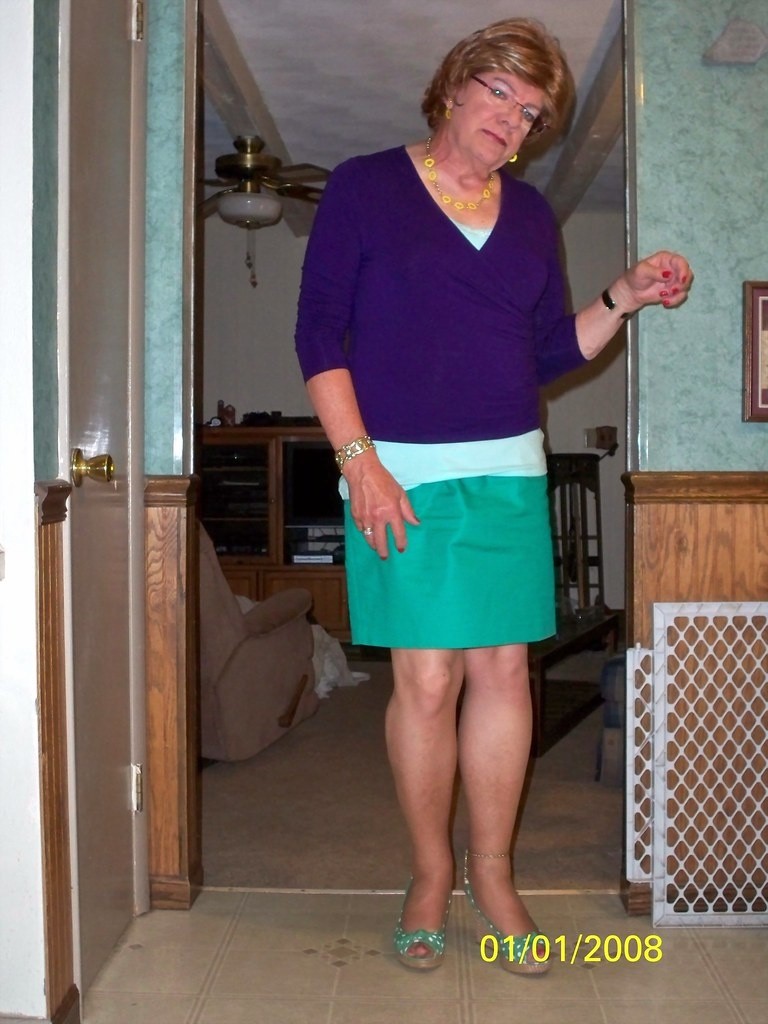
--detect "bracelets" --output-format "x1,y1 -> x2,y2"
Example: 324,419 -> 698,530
335,435 -> 376,474
602,288 -> 637,319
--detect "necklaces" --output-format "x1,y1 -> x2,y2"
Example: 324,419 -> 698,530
424,136 -> 495,210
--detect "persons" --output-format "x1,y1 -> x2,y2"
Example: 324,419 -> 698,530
293,17 -> 695,975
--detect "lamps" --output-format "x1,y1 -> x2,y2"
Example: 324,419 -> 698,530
214,134 -> 285,288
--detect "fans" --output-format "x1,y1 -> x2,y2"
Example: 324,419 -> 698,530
195,135 -> 332,222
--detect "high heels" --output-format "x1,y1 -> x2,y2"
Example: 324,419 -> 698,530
392,875 -> 453,969
463,851 -> 550,974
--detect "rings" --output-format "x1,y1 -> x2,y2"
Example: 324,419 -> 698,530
363,527 -> 374,535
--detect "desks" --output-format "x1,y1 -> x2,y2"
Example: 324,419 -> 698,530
528,612 -> 622,757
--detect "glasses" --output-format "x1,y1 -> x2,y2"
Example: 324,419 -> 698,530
470,74 -> 539,125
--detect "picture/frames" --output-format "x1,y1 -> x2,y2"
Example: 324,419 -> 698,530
742,281 -> 768,424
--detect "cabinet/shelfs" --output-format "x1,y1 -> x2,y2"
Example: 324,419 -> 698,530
200,427 -> 351,643
